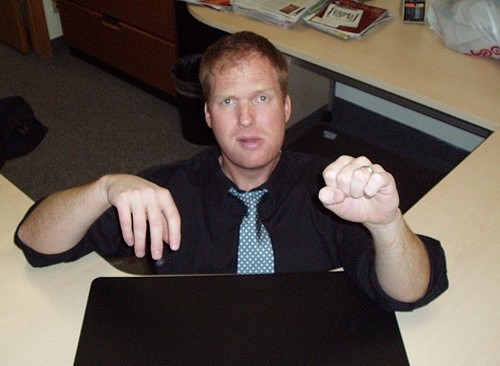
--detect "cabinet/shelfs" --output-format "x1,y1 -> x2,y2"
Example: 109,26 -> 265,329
58,0 -> 227,98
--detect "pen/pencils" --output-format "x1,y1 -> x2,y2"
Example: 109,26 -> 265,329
293,0 -> 321,15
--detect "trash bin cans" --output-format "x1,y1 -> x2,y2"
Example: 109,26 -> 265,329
169,52 -> 216,145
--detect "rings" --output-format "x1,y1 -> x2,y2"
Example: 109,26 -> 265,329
361,164 -> 374,176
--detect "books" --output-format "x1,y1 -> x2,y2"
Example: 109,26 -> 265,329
182,0 -> 395,41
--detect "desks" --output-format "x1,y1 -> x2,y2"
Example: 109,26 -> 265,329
0,0 -> 500,366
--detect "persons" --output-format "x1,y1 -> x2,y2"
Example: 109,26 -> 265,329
13,31 -> 449,311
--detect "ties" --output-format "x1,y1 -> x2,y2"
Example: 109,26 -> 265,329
229,188 -> 274,274
